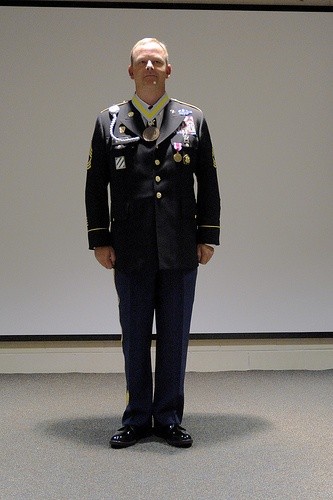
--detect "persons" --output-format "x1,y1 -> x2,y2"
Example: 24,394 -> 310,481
85,36 -> 222,448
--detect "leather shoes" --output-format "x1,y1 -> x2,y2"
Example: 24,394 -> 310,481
152,422 -> 193,447
111,422 -> 152,448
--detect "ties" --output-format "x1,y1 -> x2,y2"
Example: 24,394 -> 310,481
146,106 -> 157,127
